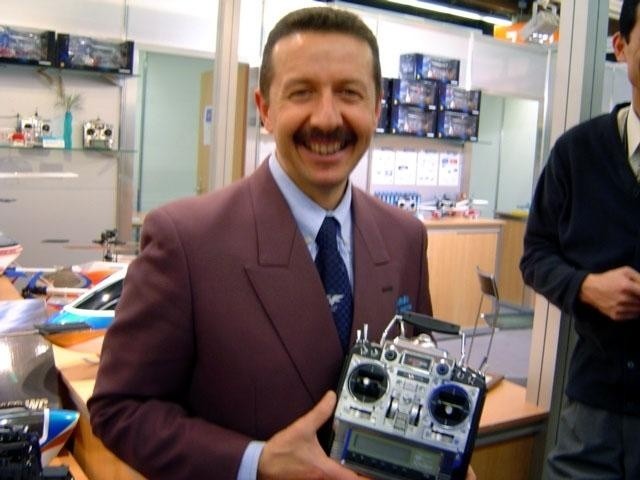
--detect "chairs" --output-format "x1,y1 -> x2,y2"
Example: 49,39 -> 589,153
466,265 -> 533,376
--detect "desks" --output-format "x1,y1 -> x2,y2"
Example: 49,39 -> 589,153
420,216 -> 503,344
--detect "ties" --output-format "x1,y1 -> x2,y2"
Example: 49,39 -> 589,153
315,217 -> 353,354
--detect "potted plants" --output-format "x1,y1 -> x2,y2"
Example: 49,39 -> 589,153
52,82 -> 81,148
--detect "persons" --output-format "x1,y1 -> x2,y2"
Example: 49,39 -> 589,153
520,0 -> 640,480
87,7 -> 476,480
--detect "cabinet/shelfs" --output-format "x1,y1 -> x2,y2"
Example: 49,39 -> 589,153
1,38 -> 146,255
